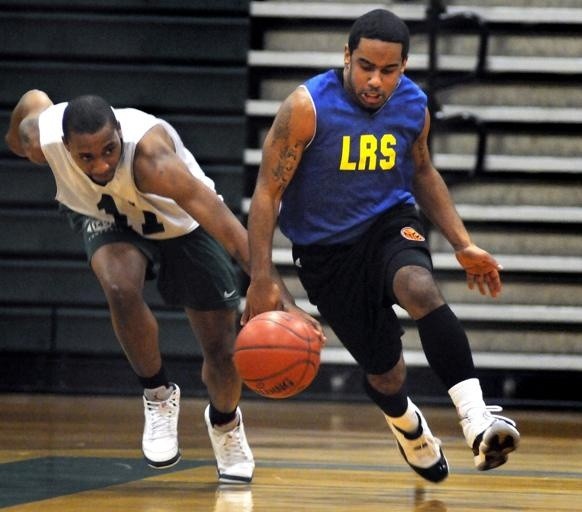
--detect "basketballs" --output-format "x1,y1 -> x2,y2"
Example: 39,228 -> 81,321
233,312 -> 322,399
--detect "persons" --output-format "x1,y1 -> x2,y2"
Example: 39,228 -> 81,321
239,9 -> 521,483
5,90 -> 327,483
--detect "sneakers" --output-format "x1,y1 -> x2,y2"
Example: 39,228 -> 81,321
202,403 -> 257,487
379,408 -> 450,486
138,383 -> 185,469
458,410 -> 521,473
